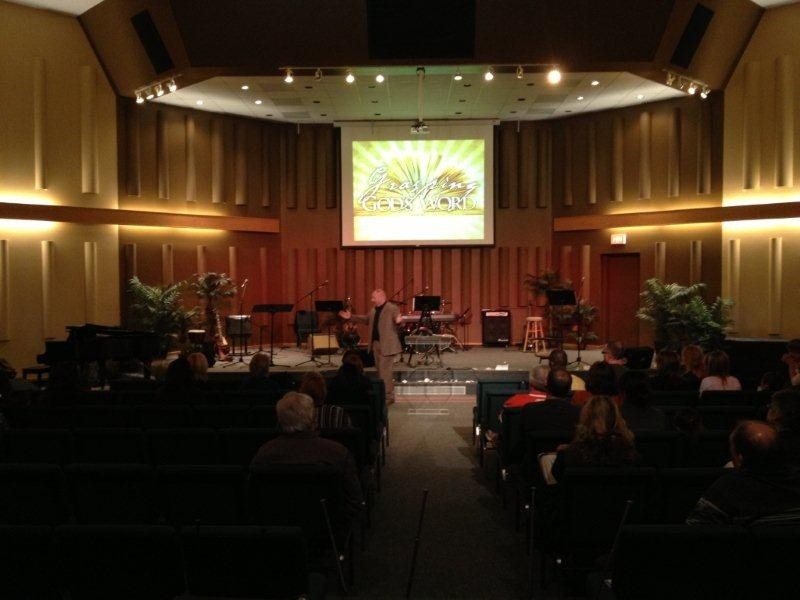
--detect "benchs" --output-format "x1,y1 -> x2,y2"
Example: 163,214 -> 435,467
22,365 -> 51,384
475,371 -> 800,600
0,367 -> 391,600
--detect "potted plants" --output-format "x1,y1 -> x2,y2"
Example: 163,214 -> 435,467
523,265 -> 573,351
665,293 -> 737,359
638,280 -> 706,364
126,271 -> 201,362
566,297 -> 600,349
194,269 -> 239,342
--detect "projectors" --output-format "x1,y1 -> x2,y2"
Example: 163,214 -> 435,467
410,121 -> 430,136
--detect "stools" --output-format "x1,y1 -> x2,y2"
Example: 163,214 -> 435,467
226,313 -> 254,357
523,316 -> 547,353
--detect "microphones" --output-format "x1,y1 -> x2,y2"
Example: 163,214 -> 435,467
241,278 -> 248,288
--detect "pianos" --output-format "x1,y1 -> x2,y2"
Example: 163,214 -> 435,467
37,323 -> 169,382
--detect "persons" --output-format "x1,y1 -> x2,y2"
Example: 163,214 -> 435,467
248,391 -> 374,567
498,336 -> 800,526
39,343 -> 376,433
338,288 -> 404,406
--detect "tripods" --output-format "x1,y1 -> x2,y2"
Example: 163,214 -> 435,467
222,284 -> 250,368
252,303 -> 294,367
565,277 -> 591,366
294,279 -> 329,369
408,295 -> 441,363
